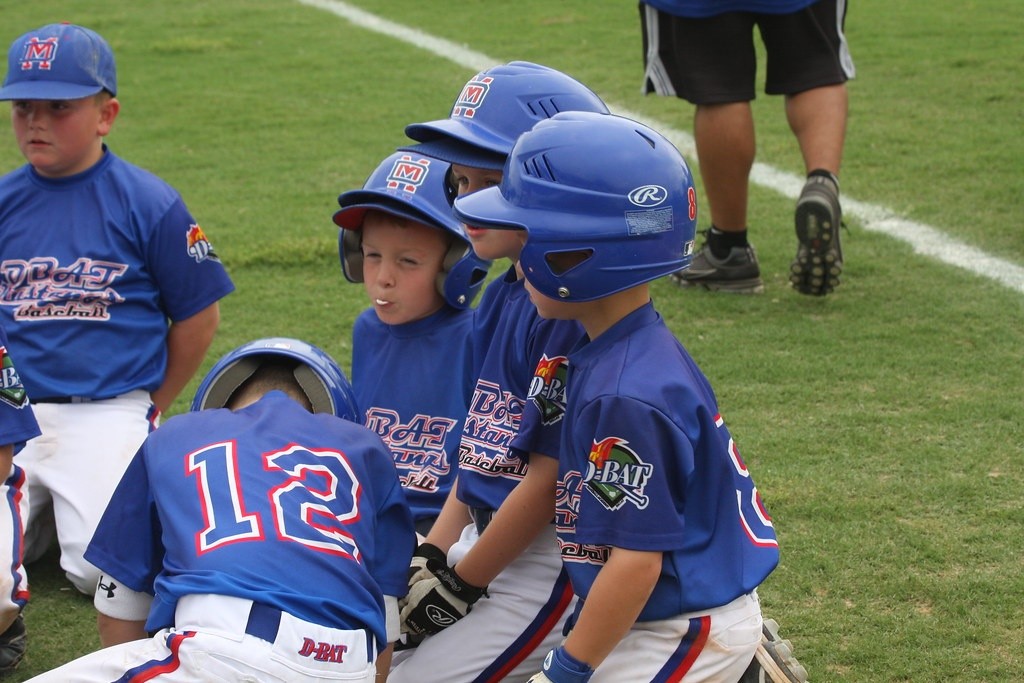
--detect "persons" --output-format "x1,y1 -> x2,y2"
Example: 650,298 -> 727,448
0,21 -> 235,596
638,0 -> 856,296
332,60 -> 812,683
0,339 -> 42,671
18,337 -> 417,683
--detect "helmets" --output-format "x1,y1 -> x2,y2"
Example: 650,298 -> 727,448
452,112 -> 697,303
337,148 -> 472,285
190,336 -> 360,423
405,60 -> 611,155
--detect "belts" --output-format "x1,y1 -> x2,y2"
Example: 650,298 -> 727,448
28,394 -> 114,404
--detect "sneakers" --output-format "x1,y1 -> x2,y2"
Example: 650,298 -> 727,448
0,616 -> 26,674
737,620 -> 812,683
789,176 -> 841,297
669,228 -> 764,294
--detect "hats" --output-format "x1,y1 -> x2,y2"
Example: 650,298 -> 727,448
332,199 -> 443,233
0,20 -> 116,101
399,128 -> 505,170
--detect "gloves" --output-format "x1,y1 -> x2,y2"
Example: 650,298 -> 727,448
525,647 -> 594,683
407,543 -> 448,591
396,558 -> 489,636
